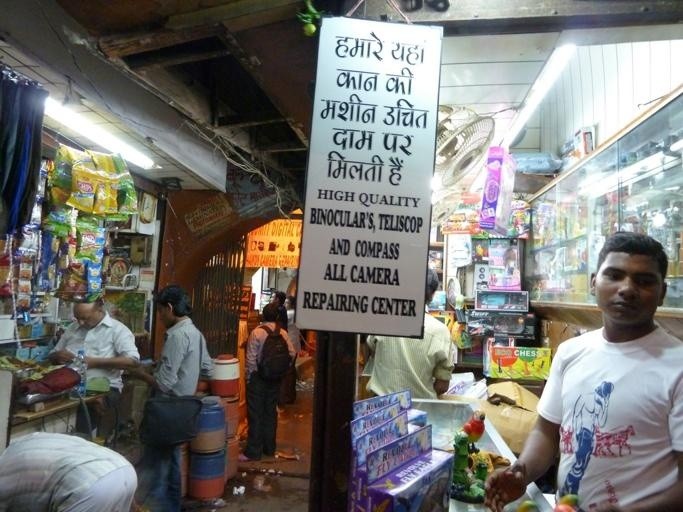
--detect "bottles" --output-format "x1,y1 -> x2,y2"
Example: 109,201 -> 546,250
67,349 -> 88,401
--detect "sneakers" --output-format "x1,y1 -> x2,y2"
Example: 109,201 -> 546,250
238,453 -> 253,461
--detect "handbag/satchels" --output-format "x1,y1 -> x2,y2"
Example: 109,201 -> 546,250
143,397 -> 203,444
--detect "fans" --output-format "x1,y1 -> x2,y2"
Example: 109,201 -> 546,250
433,105 -> 495,189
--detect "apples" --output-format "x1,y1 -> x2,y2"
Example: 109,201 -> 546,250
554,504 -> 577,512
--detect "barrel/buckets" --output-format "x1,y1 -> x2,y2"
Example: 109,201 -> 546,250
180,354 -> 241,499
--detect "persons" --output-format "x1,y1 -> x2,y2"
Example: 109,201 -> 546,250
129,284 -> 214,511
1,430 -> 139,512
365,265 -> 455,401
284,295 -> 302,402
240,303 -> 296,462
47,296 -> 142,450
479,229 -> 682,512
259,289 -> 290,412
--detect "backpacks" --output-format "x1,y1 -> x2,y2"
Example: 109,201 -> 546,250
258,325 -> 289,379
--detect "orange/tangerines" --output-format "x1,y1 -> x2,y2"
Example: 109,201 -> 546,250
516,501 -> 540,512
559,494 -> 580,506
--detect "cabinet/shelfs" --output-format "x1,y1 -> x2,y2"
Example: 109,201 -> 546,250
524,82 -> 683,318
